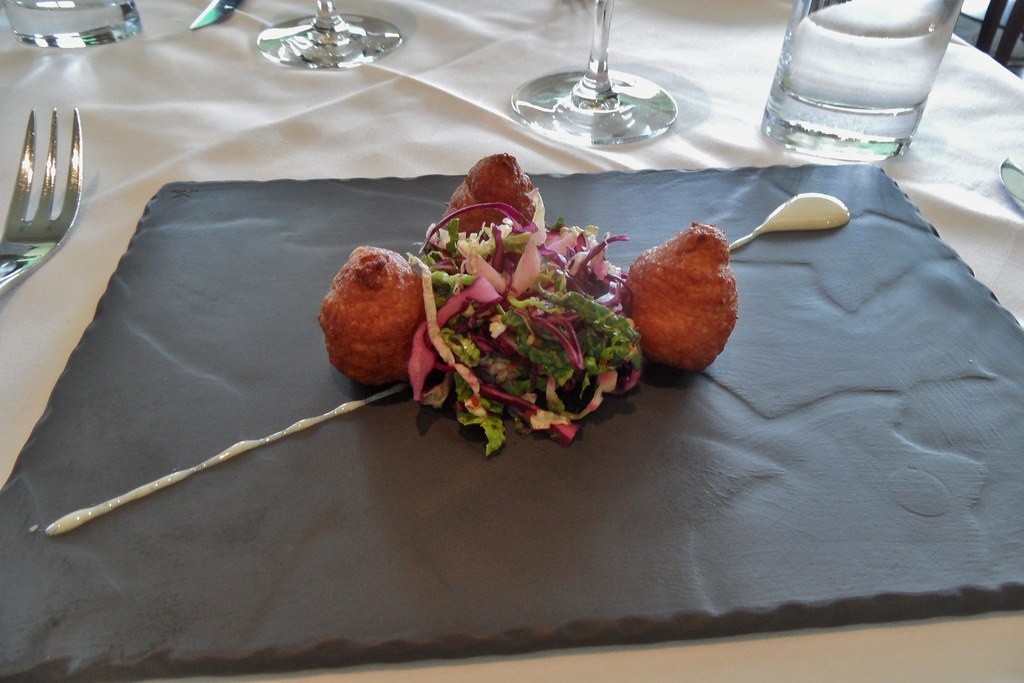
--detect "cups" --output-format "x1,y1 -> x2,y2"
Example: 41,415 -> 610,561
2,0 -> 141,49
760,0 -> 964,163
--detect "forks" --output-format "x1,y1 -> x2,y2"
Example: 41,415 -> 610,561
0,105 -> 82,297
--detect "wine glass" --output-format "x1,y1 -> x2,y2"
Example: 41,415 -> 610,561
512,0 -> 679,144
256,0 -> 403,71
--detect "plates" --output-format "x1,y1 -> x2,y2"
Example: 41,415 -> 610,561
0,156 -> 1024,680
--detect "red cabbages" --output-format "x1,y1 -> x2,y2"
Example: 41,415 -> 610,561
407,202 -> 641,447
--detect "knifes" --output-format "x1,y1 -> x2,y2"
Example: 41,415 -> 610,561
1000,156 -> 1024,206
189,0 -> 242,31
